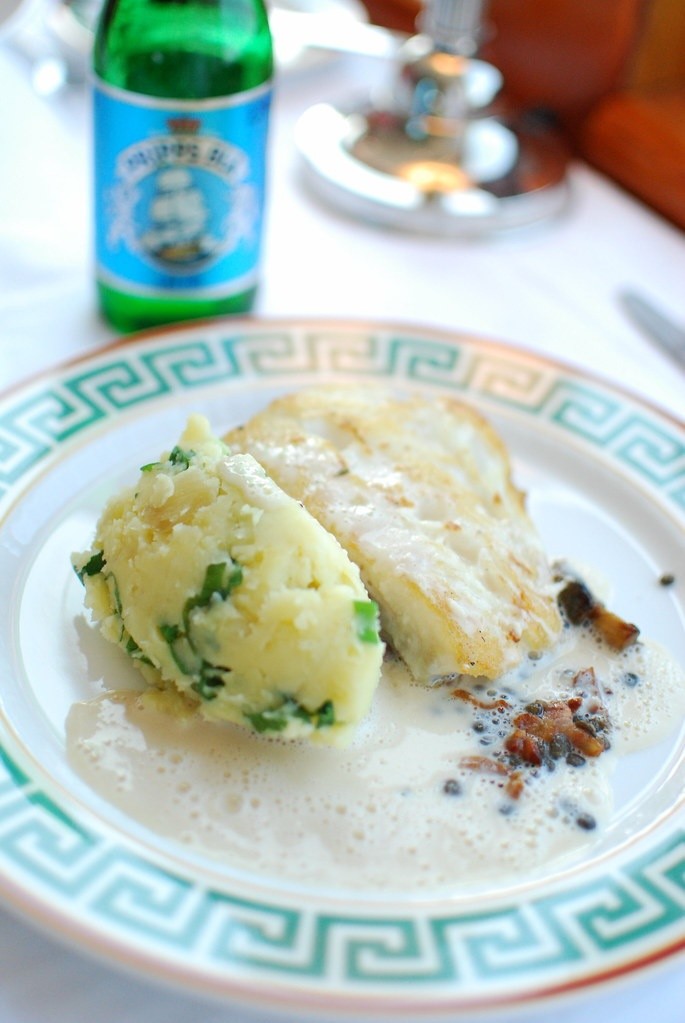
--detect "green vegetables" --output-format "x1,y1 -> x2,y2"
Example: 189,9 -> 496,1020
75,444 -> 384,736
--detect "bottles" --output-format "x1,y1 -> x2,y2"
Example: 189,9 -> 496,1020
91,0 -> 274,339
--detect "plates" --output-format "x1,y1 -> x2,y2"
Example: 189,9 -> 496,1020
0,312 -> 685,1019
